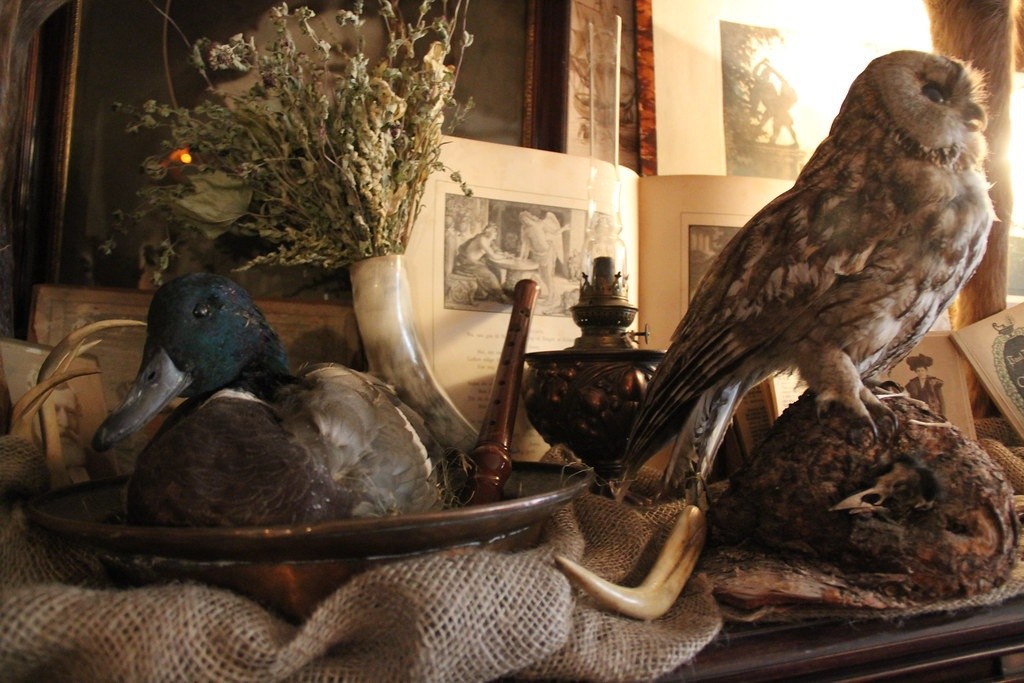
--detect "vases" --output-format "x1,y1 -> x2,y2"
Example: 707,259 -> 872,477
352,256 -> 481,458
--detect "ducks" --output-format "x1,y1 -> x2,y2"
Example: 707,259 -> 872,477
88,271 -> 448,527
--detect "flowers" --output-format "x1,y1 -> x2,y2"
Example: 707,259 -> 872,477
99,0 -> 476,295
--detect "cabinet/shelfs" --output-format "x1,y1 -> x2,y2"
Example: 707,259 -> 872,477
1,408 -> 1024,683
